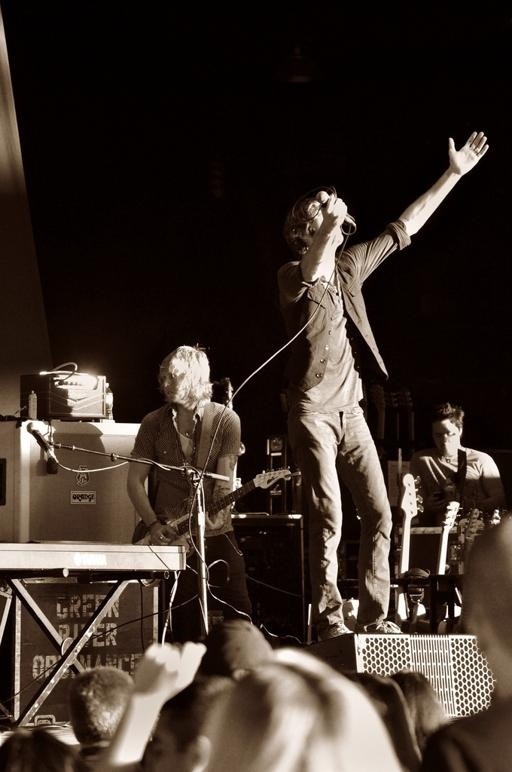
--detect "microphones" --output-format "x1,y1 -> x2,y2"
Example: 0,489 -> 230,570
27,422 -> 60,464
316,191 -> 357,227
225,381 -> 234,409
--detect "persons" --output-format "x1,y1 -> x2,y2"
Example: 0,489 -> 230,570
2,618 -> 454,771
404,399 -> 505,519
424,515 -> 511,771
271,131 -> 489,641
124,338 -> 253,629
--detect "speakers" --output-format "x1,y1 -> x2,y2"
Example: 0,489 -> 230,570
298,633 -> 497,725
0,422 -> 149,544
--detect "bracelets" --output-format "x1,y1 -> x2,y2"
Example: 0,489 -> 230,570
148,518 -> 161,530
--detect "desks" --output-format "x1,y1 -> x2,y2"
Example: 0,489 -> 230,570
1,540 -> 191,740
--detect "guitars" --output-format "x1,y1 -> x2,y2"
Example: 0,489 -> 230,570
131,466 -> 292,587
388,472 -> 502,634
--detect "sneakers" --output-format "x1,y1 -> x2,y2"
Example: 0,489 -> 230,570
355,621 -> 402,633
320,625 -> 353,639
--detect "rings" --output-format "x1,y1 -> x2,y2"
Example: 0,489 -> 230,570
474,149 -> 479,154
160,535 -> 165,540
